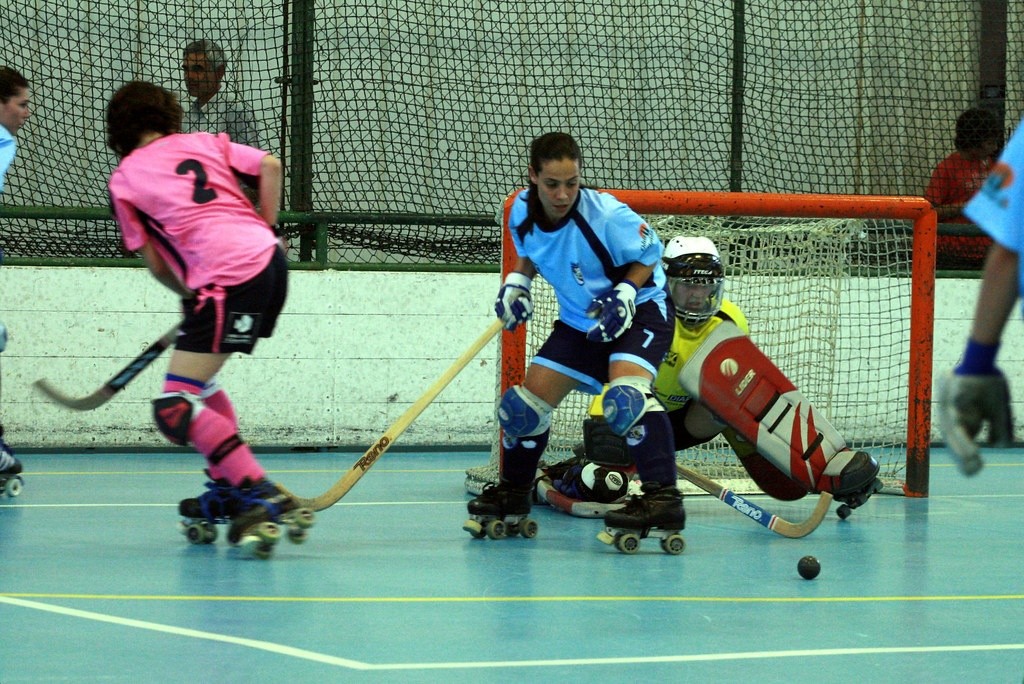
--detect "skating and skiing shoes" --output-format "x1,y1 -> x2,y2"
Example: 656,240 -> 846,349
178,473 -> 314,558
595,481 -> 686,556
536,442 -> 590,479
464,480 -> 538,541
814,446 -> 884,519
0,443 -> 24,502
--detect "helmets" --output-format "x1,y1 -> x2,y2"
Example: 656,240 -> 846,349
661,234 -> 724,331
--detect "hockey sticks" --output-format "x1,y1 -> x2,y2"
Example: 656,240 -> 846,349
30,318 -> 182,410
674,461 -> 834,540
273,318 -> 506,512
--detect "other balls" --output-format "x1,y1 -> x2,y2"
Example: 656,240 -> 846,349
797,555 -> 821,580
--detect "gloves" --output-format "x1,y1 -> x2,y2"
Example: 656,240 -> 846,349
494,272 -> 536,330
938,338 -> 1015,477
585,277 -> 639,342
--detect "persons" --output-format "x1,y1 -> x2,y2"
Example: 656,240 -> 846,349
178,41 -> 262,205
940,118 -> 1024,476
537,237 -> 884,519
462,132 -> 686,553
0,67 -> 28,500
923,107 -> 997,270
106,82 -> 316,558
